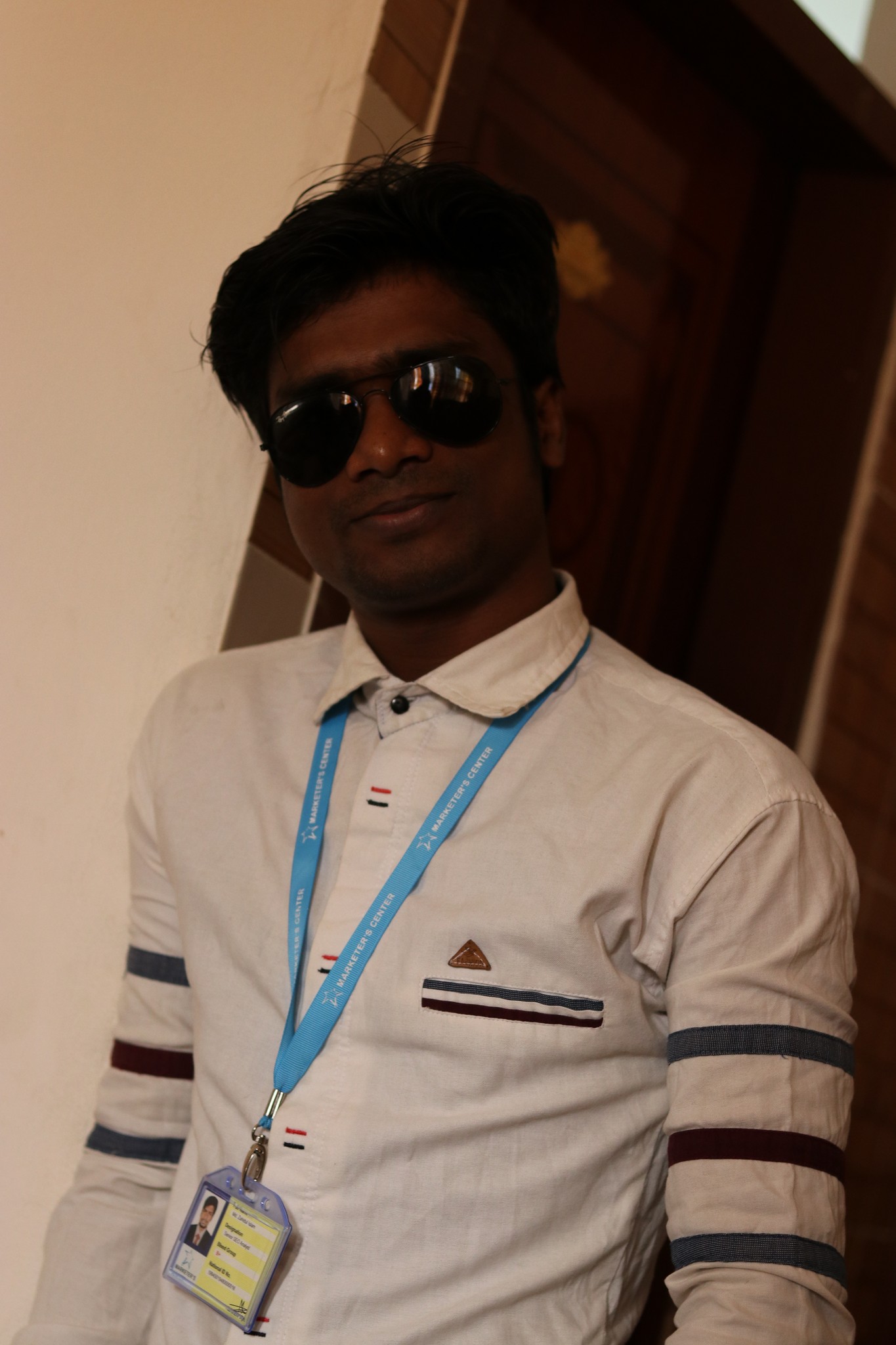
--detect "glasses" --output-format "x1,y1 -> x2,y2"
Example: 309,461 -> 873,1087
259,351 -> 513,489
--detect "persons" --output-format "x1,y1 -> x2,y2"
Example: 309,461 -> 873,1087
10,131 -> 871,1345
181,1195 -> 221,1259
